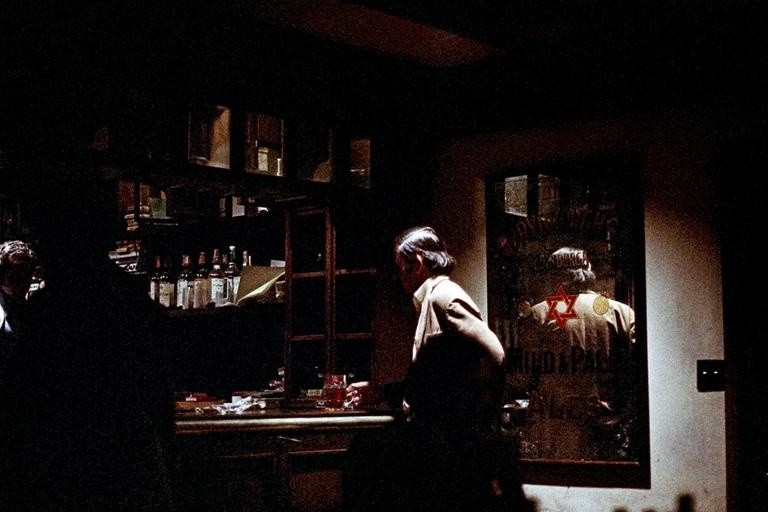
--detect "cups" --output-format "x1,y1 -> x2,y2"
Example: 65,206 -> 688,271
322,372 -> 352,412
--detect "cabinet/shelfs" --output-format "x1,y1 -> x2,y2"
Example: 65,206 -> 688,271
270,193 -> 377,406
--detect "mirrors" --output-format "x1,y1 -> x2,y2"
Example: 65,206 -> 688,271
485,144 -> 651,490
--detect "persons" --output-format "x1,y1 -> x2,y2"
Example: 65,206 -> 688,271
1,177 -> 175,510
519,245 -> 637,459
371,224 -> 506,511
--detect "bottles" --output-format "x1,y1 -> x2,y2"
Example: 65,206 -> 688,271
493,316 -> 617,376
148,242 -> 252,311
